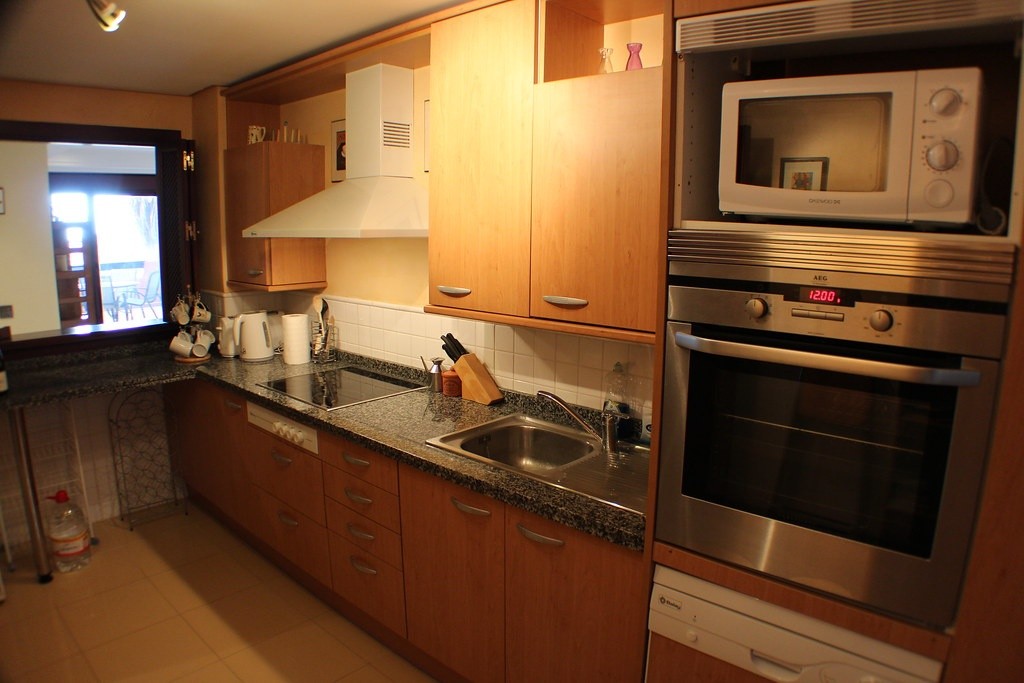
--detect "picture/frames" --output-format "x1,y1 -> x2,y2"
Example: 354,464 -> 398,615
780,157 -> 830,192
331,118 -> 346,182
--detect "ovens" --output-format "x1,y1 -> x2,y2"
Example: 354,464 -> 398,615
654,215 -> 1022,631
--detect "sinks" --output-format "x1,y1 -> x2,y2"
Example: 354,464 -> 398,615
424,412 -> 601,476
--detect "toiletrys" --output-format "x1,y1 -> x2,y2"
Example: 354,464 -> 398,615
601,360 -> 634,441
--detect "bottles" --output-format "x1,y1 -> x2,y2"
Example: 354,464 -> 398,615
48,492 -> 93,573
625,42 -> 644,72
596,47 -> 615,75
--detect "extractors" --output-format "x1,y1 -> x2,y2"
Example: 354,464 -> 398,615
239,63 -> 432,243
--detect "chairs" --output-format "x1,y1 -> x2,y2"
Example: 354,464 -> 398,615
101,271 -> 160,322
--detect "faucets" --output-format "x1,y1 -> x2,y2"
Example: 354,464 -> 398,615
536,390 -> 603,444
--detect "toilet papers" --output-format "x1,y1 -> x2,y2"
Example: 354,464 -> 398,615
281,314 -> 311,365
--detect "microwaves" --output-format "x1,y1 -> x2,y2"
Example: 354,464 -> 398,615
716,65 -> 997,230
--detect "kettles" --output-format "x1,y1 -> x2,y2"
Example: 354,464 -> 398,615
232,310 -> 275,362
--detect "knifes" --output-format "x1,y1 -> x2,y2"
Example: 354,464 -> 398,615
438,332 -> 471,362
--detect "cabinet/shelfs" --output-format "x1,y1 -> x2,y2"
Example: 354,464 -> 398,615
222,142 -> 327,293
162,378 -> 656,683
423,0 -> 664,346
51,220 -> 97,330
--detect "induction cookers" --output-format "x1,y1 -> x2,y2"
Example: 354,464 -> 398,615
256,366 -> 431,411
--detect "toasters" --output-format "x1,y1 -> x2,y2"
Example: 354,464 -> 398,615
215,309 -> 285,358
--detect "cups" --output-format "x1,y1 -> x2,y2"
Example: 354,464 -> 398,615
169,294 -> 215,358
246,126 -> 312,144
440,371 -> 462,397
